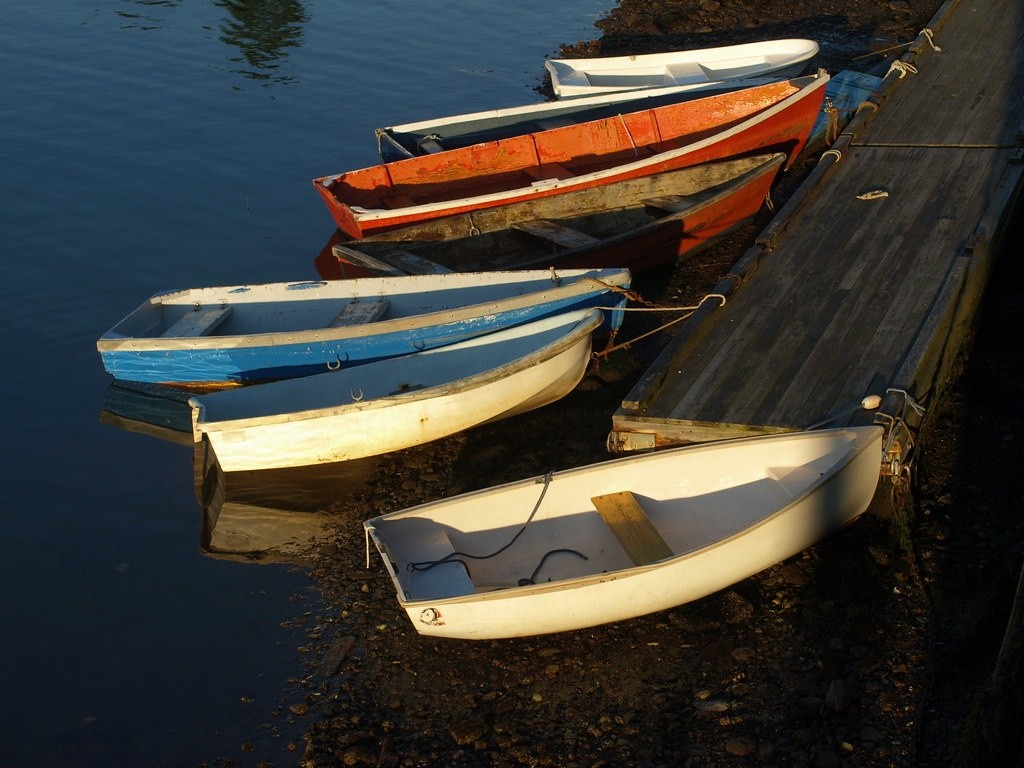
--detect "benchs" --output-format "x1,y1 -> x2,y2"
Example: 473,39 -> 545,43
392,535 -> 481,601
159,306 -> 233,338
383,195 -> 421,209
555,70 -> 592,87
535,116 -> 579,130
588,496 -> 673,565
642,195 -> 700,212
513,221 -> 598,249
329,299 -> 389,330
523,164 -> 579,182
415,138 -> 445,155
667,65 -> 711,86
378,251 -> 456,275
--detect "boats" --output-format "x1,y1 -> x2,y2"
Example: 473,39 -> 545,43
542,37 -> 820,106
191,472 -> 356,570
93,264 -> 633,387
372,73 -> 780,162
327,150 -> 784,301
97,379 -> 200,452
187,305 -> 606,478
312,69 -> 833,244
368,424 -> 887,648
